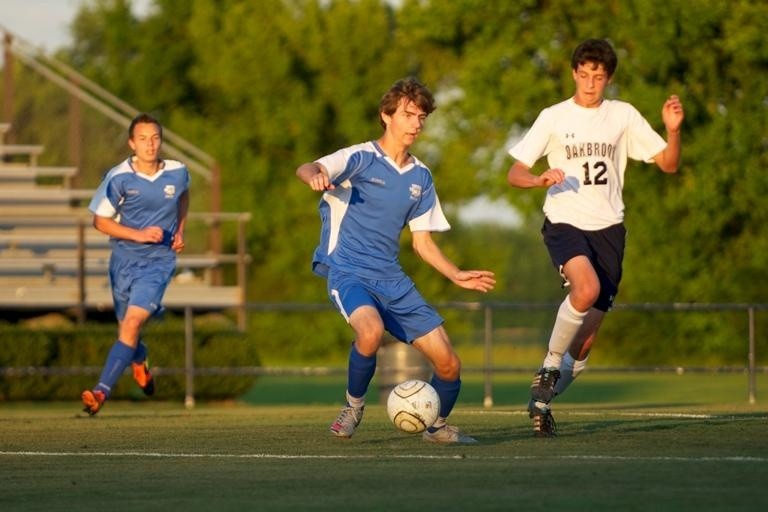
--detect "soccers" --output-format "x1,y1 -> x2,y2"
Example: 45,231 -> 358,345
386,380 -> 442,433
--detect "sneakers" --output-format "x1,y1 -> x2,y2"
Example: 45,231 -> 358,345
81,390 -> 106,416
132,355 -> 154,397
527,400 -> 557,438
530,368 -> 560,404
330,407 -> 363,439
423,425 -> 480,443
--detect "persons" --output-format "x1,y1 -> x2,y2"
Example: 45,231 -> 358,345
506,37 -> 684,438
293,79 -> 497,448
81,113 -> 192,415
